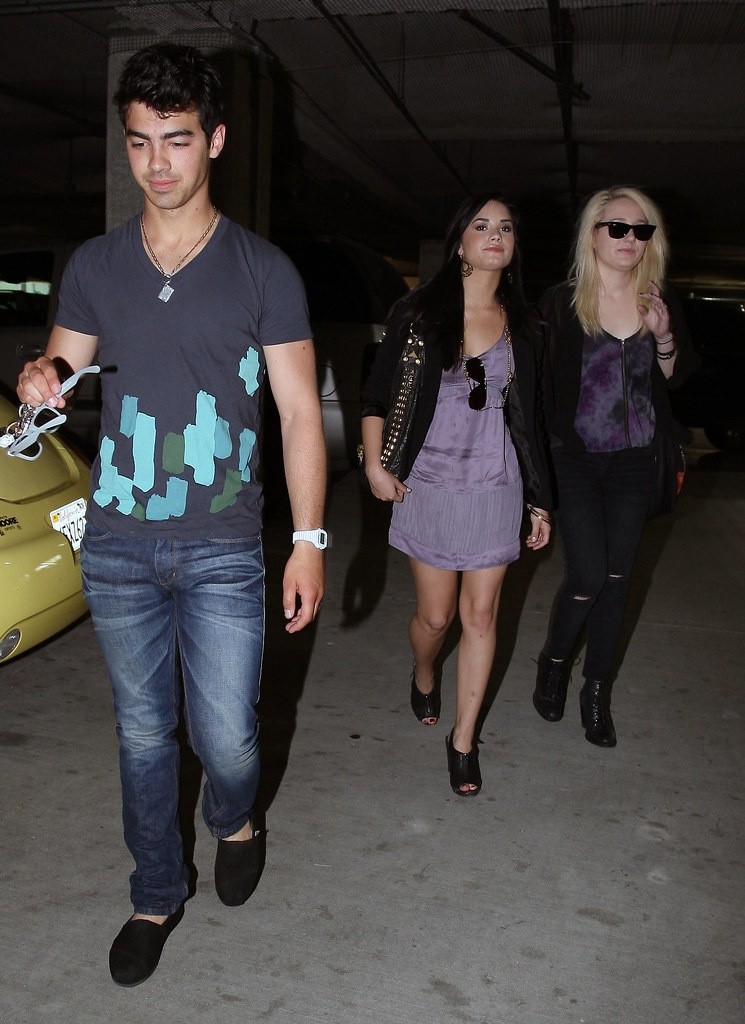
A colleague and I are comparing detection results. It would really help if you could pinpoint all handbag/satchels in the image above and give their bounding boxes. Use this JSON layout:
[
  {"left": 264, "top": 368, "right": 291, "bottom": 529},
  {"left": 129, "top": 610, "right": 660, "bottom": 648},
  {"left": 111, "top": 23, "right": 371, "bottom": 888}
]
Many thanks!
[{"left": 357, "top": 309, "right": 428, "bottom": 488}]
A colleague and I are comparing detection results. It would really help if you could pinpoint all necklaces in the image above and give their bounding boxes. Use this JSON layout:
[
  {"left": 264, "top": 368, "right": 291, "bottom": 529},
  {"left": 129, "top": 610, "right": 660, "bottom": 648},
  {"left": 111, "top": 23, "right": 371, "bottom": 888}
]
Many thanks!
[
  {"left": 460, "top": 302, "right": 512, "bottom": 412},
  {"left": 140, "top": 203, "right": 219, "bottom": 303}
]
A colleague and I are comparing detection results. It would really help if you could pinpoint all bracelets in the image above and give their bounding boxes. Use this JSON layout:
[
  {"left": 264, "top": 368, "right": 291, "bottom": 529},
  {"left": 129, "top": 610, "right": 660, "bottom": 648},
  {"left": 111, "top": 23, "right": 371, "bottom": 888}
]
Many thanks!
[
  {"left": 654, "top": 333, "right": 676, "bottom": 360},
  {"left": 529, "top": 505, "right": 550, "bottom": 524}
]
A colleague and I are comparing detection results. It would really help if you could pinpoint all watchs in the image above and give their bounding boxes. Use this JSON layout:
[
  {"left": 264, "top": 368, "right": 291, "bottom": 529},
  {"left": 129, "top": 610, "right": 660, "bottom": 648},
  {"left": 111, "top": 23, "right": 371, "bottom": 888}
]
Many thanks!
[{"left": 292, "top": 529, "right": 329, "bottom": 550}]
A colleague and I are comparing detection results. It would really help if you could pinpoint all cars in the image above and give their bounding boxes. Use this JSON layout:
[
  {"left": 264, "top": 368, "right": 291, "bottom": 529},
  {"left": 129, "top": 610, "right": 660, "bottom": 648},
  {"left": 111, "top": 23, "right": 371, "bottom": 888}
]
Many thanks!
[
  {"left": 1, "top": 395, "right": 93, "bottom": 664},
  {"left": 539, "top": 275, "right": 744, "bottom": 450}
]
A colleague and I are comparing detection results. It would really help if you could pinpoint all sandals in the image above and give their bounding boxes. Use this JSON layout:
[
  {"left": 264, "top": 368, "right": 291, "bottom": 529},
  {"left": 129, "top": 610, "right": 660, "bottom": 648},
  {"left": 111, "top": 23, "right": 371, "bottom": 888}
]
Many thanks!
[
  {"left": 410, "top": 663, "right": 441, "bottom": 726},
  {"left": 445, "top": 726, "right": 483, "bottom": 800}
]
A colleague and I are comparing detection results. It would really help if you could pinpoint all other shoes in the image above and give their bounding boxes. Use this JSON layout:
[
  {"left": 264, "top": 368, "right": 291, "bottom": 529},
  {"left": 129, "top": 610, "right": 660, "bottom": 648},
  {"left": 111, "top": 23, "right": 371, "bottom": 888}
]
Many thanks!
[
  {"left": 533, "top": 650, "right": 574, "bottom": 723},
  {"left": 579, "top": 680, "right": 618, "bottom": 749},
  {"left": 109, "top": 901, "right": 186, "bottom": 989},
  {"left": 214, "top": 816, "right": 267, "bottom": 907}
]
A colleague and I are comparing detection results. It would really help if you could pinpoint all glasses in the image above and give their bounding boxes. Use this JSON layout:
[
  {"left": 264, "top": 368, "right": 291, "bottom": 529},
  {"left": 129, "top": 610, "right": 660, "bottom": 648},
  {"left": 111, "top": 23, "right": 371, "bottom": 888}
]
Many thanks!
[
  {"left": 7, "top": 363, "right": 119, "bottom": 461},
  {"left": 595, "top": 221, "right": 657, "bottom": 242},
  {"left": 465, "top": 357, "right": 488, "bottom": 411}
]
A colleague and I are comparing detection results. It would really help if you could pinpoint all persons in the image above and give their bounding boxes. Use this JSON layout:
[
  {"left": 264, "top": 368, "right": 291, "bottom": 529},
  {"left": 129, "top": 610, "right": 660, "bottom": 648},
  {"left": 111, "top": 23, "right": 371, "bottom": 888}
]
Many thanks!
[
  {"left": 17, "top": 41, "right": 330, "bottom": 987},
  {"left": 359, "top": 189, "right": 554, "bottom": 798},
  {"left": 538, "top": 185, "right": 686, "bottom": 749}
]
